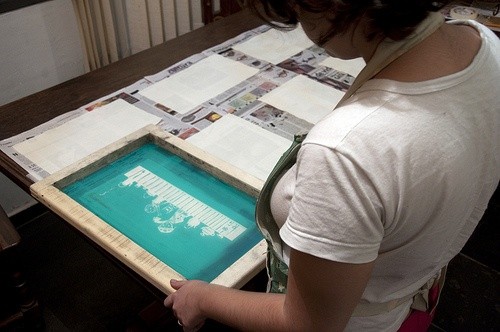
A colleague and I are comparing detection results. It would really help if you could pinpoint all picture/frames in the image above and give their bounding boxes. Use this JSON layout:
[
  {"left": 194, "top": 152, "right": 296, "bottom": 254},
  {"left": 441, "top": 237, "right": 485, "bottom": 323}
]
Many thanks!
[{"left": 30, "top": 122, "right": 270, "bottom": 302}]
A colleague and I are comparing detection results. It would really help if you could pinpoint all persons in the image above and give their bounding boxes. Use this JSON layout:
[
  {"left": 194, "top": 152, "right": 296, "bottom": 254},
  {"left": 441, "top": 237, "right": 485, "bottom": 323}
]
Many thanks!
[{"left": 165, "top": 0, "right": 500, "bottom": 332}]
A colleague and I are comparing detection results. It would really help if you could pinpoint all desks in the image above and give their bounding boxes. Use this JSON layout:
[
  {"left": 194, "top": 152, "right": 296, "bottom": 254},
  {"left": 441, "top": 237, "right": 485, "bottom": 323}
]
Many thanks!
[{"left": 0, "top": 1, "right": 368, "bottom": 330}]
